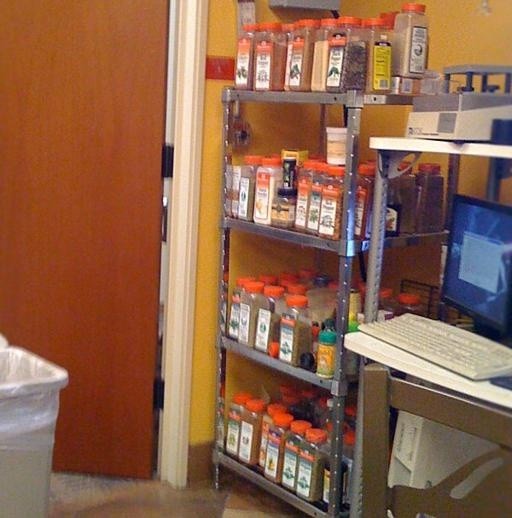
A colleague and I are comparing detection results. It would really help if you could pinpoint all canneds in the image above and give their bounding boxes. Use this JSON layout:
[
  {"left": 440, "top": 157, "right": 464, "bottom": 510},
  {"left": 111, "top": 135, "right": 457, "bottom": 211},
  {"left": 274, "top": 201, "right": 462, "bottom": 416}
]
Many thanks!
[{"left": 271, "top": 186, "right": 296, "bottom": 230}]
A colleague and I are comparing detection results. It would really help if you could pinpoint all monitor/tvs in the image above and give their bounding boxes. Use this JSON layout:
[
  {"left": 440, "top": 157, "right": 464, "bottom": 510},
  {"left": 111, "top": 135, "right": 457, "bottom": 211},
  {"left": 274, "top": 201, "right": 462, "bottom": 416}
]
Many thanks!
[{"left": 441, "top": 194, "right": 512, "bottom": 340}]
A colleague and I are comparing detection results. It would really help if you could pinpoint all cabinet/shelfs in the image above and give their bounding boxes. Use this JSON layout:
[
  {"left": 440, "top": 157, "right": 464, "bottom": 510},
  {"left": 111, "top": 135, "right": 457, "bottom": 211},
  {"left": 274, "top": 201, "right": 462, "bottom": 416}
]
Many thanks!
[
  {"left": 210, "top": 86, "right": 460, "bottom": 517},
  {"left": 351, "top": 135, "right": 511, "bottom": 517}
]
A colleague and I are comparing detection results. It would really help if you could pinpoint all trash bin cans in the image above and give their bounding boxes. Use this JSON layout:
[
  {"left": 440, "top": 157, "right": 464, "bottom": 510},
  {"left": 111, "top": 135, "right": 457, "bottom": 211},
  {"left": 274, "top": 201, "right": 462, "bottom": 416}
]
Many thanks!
[{"left": 1, "top": 345, "right": 70, "bottom": 518}]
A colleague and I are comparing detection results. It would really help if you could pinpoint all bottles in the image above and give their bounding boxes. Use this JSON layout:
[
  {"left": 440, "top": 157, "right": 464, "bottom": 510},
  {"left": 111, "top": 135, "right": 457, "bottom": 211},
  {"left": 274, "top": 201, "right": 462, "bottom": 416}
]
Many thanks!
[
  {"left": 225, "top": 270, "right": 424, "bottom": 510},
  {"left": 236, "top": 2, "right": 445, "bottom": 97},
  {"left": 228, "top": 127, "right": 445, "bottom": 239}
]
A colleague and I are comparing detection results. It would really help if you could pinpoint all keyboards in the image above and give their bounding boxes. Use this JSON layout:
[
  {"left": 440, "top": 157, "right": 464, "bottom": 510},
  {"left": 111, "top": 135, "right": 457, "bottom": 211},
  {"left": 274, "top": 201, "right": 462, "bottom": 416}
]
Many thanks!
[{"left": 358, "top": 312, "right": 512, "bottom": 380}]
